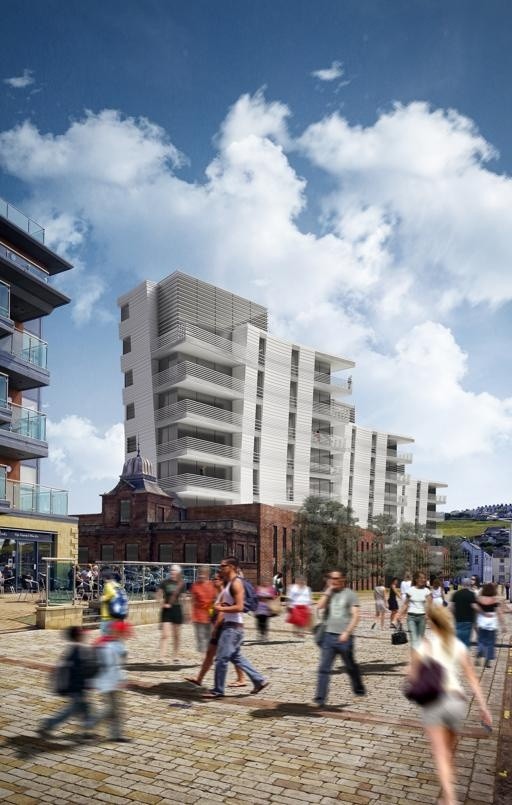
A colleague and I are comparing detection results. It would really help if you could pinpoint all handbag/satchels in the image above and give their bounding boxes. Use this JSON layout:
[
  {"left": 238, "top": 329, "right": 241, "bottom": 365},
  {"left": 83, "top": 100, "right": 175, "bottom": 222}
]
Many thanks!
[
  {"left": 402, "top": 659, "right": 447, "bottom": 707},
  {"left": 311, "top": 598, "right": 331, "bottom": 646},
  {"left": 390, "top": 621, "right": 408, "bottom": 645}
]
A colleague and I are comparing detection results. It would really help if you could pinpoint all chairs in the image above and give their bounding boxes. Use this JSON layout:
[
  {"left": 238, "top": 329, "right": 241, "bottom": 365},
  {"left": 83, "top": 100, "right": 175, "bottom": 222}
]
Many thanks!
[{"left": 0, "top": 565, "right": 184, "bottom": 602}]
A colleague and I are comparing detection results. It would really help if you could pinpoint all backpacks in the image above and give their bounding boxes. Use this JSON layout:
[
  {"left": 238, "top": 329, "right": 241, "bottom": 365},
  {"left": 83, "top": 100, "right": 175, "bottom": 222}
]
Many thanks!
[
  {"left": 109, "top": 587, "right": 130, "bottom": 620},
  {"left": 242, "top": 579, "right": 258, "bottom": 613}
]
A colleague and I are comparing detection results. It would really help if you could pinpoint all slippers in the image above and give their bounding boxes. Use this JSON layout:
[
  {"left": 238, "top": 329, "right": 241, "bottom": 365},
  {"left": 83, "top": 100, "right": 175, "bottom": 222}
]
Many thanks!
[
  {"left": 199, "top": 691, "right": 224, "bottom": 699},
  {"left": 184, "top": 677, "right": 201, "bottom": 687},
  {"left": 227, "top": 682, "right": 246, "bottom": 687},
  {"left": 250, "top": 682, "right": 270, "bottom": 695}
]
